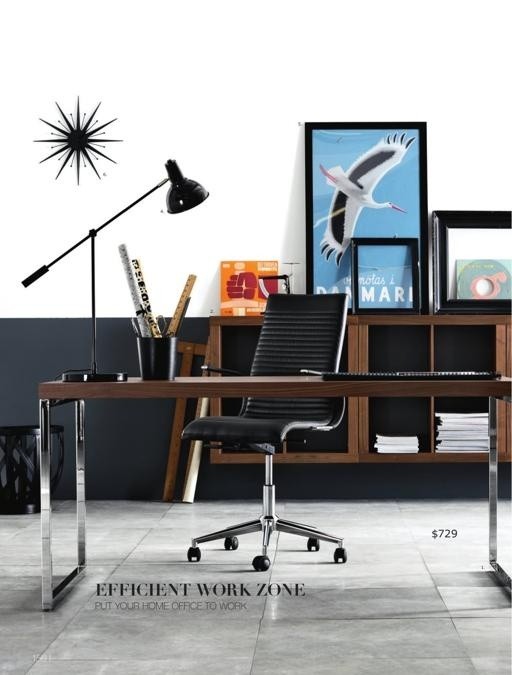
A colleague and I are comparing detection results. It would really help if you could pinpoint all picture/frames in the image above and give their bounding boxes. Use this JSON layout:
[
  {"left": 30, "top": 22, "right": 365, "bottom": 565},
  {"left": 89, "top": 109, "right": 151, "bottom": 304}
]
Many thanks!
[{"left": 304, "top": 119, "right": 512, "bottom": 316}]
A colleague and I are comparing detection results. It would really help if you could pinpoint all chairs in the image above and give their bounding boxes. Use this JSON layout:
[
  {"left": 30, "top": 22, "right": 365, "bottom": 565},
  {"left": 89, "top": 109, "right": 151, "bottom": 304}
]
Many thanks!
[{"left": 180, "top": 294, "right": 350, "bottom": 571}]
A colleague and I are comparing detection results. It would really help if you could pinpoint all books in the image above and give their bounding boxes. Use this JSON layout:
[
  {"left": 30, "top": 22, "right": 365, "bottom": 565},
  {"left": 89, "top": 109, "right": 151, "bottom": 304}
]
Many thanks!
[
  {"left": 433, "top": 412, "right": 490, "bottom": 453},
  {"left": 373, "top": 433, "right": 421, "bottom": 454}
]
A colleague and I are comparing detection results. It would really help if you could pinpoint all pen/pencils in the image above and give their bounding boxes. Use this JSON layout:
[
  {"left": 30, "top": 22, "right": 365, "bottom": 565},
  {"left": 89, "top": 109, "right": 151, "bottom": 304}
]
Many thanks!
[{"left": 130, "top": 317, "right": 140, "bottom": 337}]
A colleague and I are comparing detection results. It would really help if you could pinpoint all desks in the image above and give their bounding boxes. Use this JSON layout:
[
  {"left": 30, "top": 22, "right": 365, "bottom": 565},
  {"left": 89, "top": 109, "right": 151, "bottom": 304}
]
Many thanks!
[{"left": 35, "top": 373, "right": 511, "bottom": 611}]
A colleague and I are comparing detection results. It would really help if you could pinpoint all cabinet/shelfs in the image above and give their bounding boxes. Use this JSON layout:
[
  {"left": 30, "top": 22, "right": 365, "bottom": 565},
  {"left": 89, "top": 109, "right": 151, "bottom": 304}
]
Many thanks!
[{"left": 208, "top": 312, "right": 511, "bottom": 463}]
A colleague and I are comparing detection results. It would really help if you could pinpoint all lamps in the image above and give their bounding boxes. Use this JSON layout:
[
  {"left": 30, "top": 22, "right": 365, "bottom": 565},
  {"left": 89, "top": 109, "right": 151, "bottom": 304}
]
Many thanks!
[{"left": 22, "top": 157, "right": 210, "bottom": 381}]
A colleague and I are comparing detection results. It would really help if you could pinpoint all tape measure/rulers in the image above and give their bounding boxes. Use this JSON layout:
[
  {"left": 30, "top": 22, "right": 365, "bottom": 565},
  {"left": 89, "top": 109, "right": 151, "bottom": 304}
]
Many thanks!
[{"left": 167, "top": 274, "right": 196, "bottom": 336}]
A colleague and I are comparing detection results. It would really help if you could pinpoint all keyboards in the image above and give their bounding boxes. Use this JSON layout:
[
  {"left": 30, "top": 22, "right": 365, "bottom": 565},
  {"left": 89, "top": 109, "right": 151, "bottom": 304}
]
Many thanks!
[{"left": 323, "top": 371, "right": 497, "bottom": 380}]
